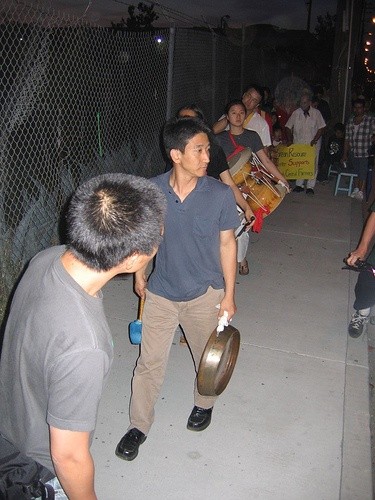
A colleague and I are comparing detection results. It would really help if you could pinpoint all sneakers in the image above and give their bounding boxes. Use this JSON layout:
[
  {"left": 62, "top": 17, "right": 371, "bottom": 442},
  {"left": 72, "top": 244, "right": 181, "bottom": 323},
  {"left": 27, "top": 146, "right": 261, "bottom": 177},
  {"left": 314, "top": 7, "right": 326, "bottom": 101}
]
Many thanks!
[{"left": 347, "top": 310, "right": 370, "bottom": 338}]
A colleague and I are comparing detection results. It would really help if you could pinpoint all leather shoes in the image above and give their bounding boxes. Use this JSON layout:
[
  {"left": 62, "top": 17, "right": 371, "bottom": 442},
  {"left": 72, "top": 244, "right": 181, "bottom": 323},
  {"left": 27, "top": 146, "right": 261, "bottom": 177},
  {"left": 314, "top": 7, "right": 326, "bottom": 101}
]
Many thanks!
[
  {"left": 116, "top": 428, "right": 146, "bottom": 460},
  {"left": 186, "top": 406, "right": 213, "bottom": 431}
]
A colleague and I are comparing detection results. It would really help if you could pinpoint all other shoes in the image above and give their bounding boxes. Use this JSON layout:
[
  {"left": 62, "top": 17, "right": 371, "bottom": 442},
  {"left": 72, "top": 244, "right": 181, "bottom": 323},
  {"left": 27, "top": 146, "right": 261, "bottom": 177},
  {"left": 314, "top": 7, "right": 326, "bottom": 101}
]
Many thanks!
[
  {"left": 293, "top": 187, "right": 303, "bottom": 192},
  {"left": 306, "top": 188, "right": 314, "bottom": 195},
  {"left": 351, "top": 189, "right": 364, "bottom": 200}
]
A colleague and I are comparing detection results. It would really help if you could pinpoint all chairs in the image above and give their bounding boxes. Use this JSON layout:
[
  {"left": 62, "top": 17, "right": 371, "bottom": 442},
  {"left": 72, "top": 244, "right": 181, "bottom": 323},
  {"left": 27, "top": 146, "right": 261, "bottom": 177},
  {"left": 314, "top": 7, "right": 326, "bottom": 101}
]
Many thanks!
[{"left": 324, "top": 160, "right": 357, "bottom": 195}]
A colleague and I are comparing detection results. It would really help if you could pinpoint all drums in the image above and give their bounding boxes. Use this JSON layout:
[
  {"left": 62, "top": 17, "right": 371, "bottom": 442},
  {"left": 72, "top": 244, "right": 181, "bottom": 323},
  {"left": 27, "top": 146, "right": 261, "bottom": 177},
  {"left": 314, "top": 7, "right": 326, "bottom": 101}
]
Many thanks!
[{"left": 228, "top": 148, "right": 287, "bottom": 219}]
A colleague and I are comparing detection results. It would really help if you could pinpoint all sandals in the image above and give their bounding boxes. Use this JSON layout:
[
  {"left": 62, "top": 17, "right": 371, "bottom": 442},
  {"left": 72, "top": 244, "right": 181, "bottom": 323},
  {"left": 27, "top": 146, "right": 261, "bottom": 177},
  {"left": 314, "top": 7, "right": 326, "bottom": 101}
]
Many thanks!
[{"left": 238, "top": 261, "right": 248, "bottom": 274}]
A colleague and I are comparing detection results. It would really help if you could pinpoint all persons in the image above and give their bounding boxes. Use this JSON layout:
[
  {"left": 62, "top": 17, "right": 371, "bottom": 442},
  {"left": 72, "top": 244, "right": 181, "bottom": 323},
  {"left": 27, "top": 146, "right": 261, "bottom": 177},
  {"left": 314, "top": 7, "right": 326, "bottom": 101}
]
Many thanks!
[
  {"left": 257, "top": 108, "right": 272, "bottom": 137},
  {"left": 347, "top": 200, "right": 375, "bottom": 338},
  {"left": 318, "top": 122, "right": 344, "bottom": 184},
  {"left": 311, "top": 86, "right": 331, "bottom": 124},
  {"left": 116, "top": 116, "right": 241, "bottom": 461},
  {"left": 215, "top": 100, "right": 292, "bottom": 275},
  {"left": 178, "top": 102, "right": 255, "bottom": 231},
  {"left": 213, "top": 86, "right": 272, "bottom": 156},
  {"left": 0, "top": 172, "right": 166, "bottom": 499},
  {"left": 285, "top": 93, "right": 326, "bottom": 195},
  {"left": 343, "top": 98, "right": 375, "bottom": 202}
]
